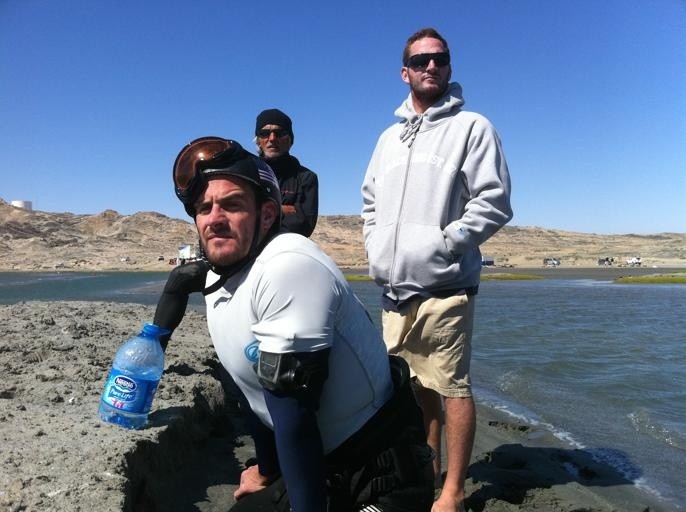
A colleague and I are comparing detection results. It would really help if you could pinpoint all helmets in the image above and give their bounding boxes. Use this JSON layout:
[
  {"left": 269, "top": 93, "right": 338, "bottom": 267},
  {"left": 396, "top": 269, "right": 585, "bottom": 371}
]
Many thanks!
[{"left": 185, "top": 151, "right": 282, "bottom": 241}]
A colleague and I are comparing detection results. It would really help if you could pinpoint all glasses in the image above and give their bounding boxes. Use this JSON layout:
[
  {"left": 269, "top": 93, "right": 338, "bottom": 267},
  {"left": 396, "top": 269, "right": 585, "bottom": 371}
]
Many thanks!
[
  {"left": 257, "top": 128, "right": 287, "bottom": 138},
  {"left": 173, "top": 136, "right": 243, "bottom": 206},
  {"left": 407, "top": 53, "right": 450, "bottom": 69}
]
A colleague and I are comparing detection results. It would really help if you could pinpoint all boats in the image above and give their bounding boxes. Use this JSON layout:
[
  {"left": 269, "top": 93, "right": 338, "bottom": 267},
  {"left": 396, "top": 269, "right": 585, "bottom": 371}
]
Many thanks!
[
  {"left": 346, "top": 274, "right": 547, "bottom": 281},
  {"left": 613, "top": 276, "right": 686, "bottom": 284}
]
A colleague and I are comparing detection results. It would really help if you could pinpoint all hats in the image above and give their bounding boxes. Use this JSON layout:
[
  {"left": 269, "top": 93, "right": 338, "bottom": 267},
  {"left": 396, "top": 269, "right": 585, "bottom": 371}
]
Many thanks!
[{"left": 255, "top": 109, "right": 294, "bottom": 144}]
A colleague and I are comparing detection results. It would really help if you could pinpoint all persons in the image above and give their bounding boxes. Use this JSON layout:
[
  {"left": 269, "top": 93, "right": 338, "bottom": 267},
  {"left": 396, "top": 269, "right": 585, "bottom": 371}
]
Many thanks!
[
  {"left": 142, "top": 107, "right": 320, "bottom": 353},
  {"left": 171, "top": 134, "right": 438, "bottom": 511},
  {"left": 359, "top": 27, "right": 516, "bottom": 511}
]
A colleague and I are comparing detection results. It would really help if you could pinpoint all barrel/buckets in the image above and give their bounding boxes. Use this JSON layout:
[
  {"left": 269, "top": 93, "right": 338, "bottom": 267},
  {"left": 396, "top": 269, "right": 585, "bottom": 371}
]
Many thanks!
[{"left": 97, "top": 322, "right": 171, "bottom": 429}]
